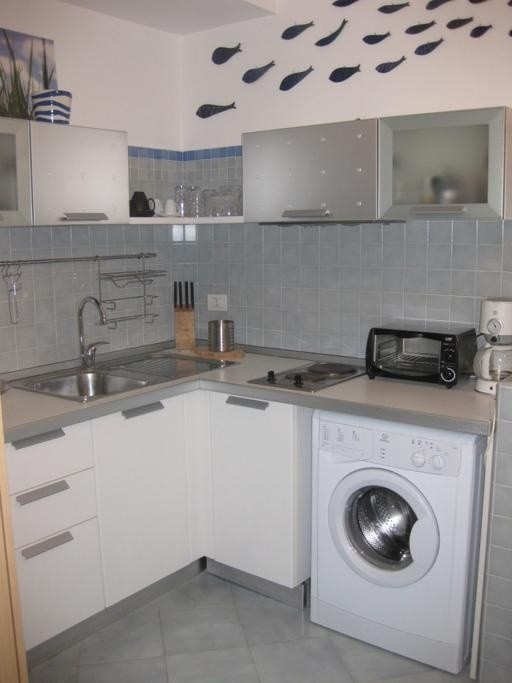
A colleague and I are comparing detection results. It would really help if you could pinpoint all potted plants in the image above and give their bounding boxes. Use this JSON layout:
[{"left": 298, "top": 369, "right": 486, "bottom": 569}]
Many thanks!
[{"left": 31, "top": 63, "right": 72, "bottom": 125}]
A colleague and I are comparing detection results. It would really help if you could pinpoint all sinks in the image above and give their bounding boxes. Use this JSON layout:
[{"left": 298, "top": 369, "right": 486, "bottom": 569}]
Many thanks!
[
  {"left": 106, "top": 352, "right": 237, "bottom": 384},
  {"left": 6, "top": 367, "right": 151, "bottom": 404}
]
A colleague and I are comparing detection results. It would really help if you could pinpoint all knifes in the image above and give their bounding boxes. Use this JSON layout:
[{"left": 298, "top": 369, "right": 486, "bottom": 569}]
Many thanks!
[
  {"left": 174, "top": 281, "right": 177, "bottom": 308},
  {"left": 185, "top": 282, "right": 188, "bottom": 307},
  {"left": 179, "top": 282, "right": 182, "bottom": 307},
  {"left": 190, "top": 282, "right": 194, "bottom": 308}
]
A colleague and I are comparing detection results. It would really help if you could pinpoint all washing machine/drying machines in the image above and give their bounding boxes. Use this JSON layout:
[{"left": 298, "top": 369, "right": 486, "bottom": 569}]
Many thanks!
[{"left": 310, "top": 409, "right": 487, "bottom": 674}]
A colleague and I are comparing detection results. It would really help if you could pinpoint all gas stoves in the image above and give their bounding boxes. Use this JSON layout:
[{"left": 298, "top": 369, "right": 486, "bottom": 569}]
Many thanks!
[{"left": 246, "top": 362, "right": 368, "bottom": 392}]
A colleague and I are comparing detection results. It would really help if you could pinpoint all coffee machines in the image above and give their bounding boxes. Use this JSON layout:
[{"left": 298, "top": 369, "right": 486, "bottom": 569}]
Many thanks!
[{"left": 472, "top": 297, "right": 512, "bottom": 395}]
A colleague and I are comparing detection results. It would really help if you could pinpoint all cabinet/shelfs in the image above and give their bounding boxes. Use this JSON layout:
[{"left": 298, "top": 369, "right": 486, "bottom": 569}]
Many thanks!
[
  {"left": 3, "top": 420, "right": 106, "bottom": 652},
  {"left": 204, "top": 392, "right": 311, "bottom": 589},
  {"left": 97, "top": 253, "right": 168, "bottom": 329},
  {"left": 1, "top": 118, "right": 33, "bottom": 228},
  {"left": 242, "top": 118, "right": 377, "bottom": 223},
  {"left": 93, "top": 388, "right": 204, "bottom": 609},
  {"left": 29, "top": 120, "right": 130, "bottom": 226}
]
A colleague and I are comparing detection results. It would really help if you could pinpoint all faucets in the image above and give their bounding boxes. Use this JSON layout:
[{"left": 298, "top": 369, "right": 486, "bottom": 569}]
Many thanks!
[{"left": 76, "top": 295, "right": 110, "bottom": 368}]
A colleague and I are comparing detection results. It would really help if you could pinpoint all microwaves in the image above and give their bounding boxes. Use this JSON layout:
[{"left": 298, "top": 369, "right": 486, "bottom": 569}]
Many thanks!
[{"left": 365, "top": 320, "right": 476, "bottom": 389}]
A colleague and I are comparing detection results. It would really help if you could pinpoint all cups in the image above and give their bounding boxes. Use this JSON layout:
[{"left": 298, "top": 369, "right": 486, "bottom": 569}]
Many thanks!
[
  {"left": 208, "top": 320, "right": 235, "bottom": 352},
  {"left": 203, "top": 184, "right": 243, "bottom": 216},
  {"left": 149, "top": 199, "right": 164, "bottom": 216},
  {"left": 176, "top": 185, "right": 188, "bottom": 218},
  {"left": 164, "top": 199, "right": 181, "bottom": 215},
  {"left": 189, "top": 184, "right": 201, "bottom": 218},
  {"left": 130, "top": 191, "right": 155, "bottom": 213}
]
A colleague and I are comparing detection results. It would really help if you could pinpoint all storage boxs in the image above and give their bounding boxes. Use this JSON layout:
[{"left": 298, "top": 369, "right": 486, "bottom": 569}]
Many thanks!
[{"left": 378, "top": 106, "right": 512, "bottom": 222}]
